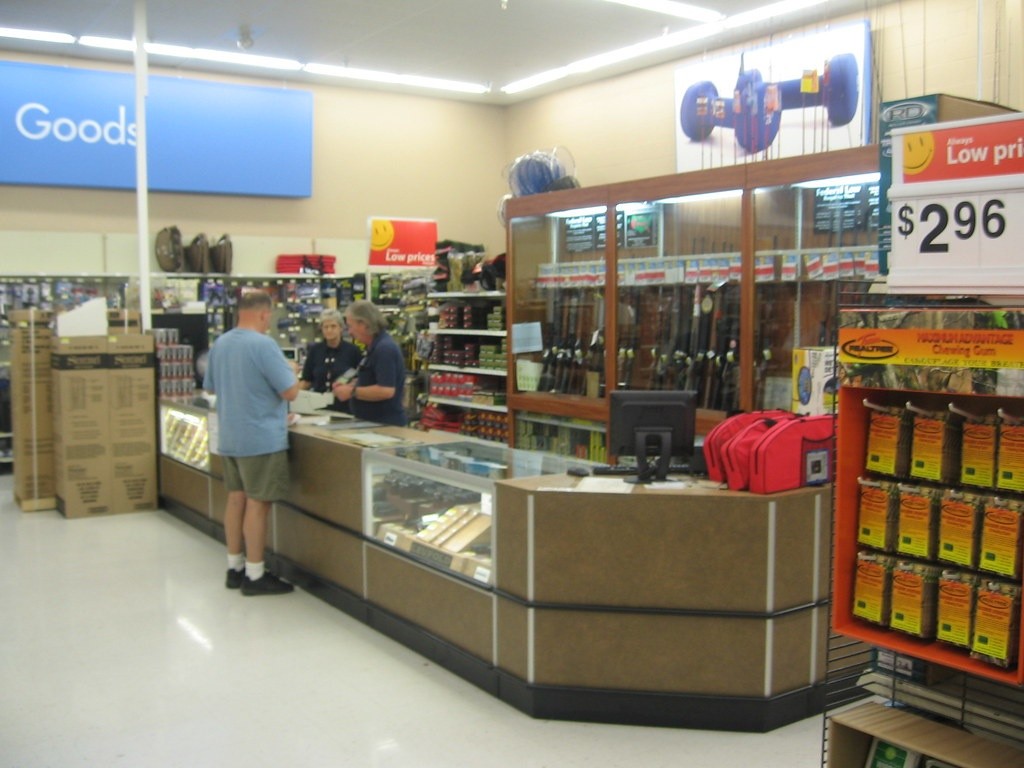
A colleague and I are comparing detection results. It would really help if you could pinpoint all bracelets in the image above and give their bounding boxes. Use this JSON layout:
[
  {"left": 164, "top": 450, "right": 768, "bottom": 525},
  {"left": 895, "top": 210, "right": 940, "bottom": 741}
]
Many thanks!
[{"left": 352, "top": 387, "right": 356, "bottom": 399}]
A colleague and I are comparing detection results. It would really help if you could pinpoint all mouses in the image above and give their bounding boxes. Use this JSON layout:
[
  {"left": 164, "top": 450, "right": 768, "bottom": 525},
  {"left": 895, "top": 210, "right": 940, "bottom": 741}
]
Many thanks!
[{"left": 567, "top": 466, "right": 589, "bottom": 476}]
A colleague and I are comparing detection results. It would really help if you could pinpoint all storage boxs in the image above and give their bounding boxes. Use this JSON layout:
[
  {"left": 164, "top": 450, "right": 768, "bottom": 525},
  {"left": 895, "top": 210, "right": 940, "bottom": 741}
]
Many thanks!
[
  {"left": 6, "top": 309, "right": 144, "bottom": 513},
  {"left": 827, "top": 703, "right": 1024, "bottom": 768},
  {"left": 48, "top": 333, "right": 160, "bottom": 519}
]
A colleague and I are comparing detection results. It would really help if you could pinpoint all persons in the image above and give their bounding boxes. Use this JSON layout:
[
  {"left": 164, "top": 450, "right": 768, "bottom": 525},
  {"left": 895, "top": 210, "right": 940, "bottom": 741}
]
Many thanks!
[
  {"left": 203, "top": 291, "right": 300, "bottom": 596},
  {"left": 332, "top": 300, "right": 405, "bottom": 427},
  {"left": 299, "top": 308, "right": 362, "bottom": 415}
]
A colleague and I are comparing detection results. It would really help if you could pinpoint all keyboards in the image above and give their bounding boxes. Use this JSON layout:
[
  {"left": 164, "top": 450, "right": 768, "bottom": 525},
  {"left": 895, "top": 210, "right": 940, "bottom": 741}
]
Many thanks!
[{"left": 592, "top": 465, "right": 695, "bottom": 475}]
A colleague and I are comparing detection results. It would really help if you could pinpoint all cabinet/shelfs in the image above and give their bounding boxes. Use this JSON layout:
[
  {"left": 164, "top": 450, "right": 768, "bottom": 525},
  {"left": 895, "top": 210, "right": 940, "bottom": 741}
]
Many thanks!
[
  {"left": 825, "top": 307, "right": 1024, "bottom": 687},
  {"left": 0, "top": 272, "right": 422, "bottom": 465},
  {"left": 424, "top": 288, "right": 509, "bottom": 414},
  {"left": 510, "top": 142, "right": 882, "bottom": 469},
  {"left": 157, "top": 394, "right": 832, "bottom": 734}
]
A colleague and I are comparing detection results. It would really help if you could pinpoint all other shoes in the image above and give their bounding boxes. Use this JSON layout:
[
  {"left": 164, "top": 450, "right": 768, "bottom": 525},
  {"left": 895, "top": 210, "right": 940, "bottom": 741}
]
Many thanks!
[
  {"left": 242, "top": 571, "right": 294, "bottom": 595},
  {"left": 227, "top": 566, "right": 278, "bottom": 589}
]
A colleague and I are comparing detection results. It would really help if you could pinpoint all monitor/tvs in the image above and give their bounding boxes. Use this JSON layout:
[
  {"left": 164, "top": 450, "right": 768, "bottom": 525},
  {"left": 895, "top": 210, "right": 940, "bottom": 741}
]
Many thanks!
[{"left": 609, "top": 389, "right": 698, "bottom": 485}]
